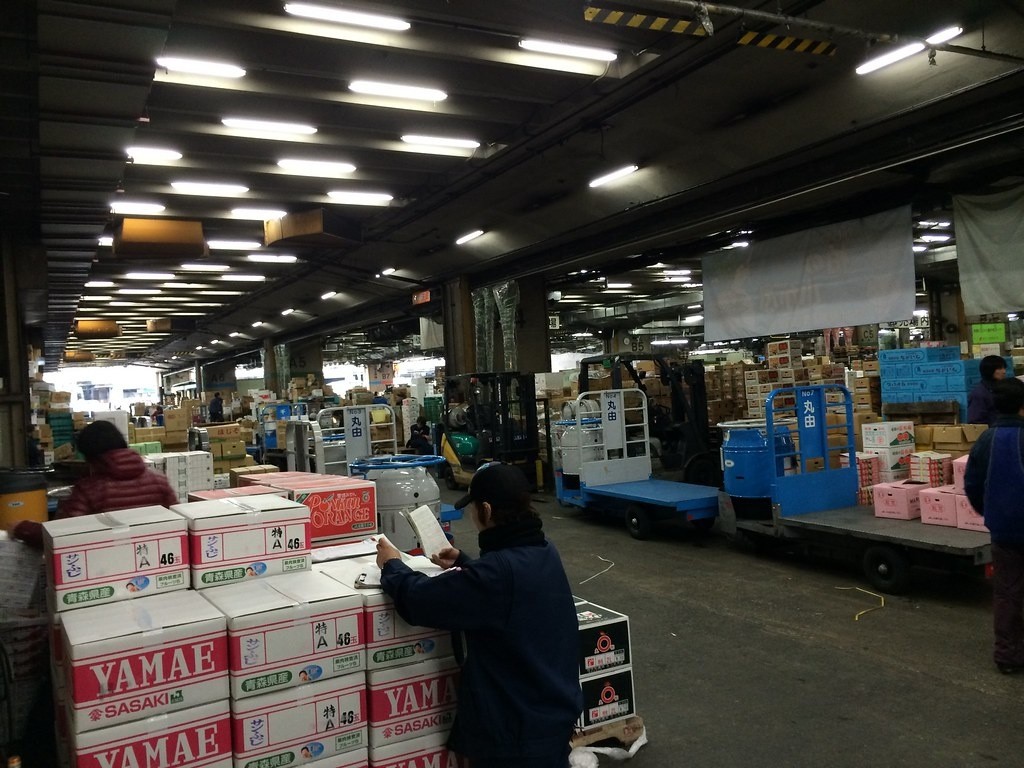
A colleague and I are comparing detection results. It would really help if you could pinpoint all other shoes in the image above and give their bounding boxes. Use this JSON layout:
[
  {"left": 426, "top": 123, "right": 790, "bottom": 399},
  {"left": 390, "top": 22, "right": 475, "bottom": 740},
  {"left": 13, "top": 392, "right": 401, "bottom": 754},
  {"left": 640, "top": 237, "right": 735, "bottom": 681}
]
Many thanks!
[{"left": 997, "top": 661, "right": 1024, "bottom": 675}]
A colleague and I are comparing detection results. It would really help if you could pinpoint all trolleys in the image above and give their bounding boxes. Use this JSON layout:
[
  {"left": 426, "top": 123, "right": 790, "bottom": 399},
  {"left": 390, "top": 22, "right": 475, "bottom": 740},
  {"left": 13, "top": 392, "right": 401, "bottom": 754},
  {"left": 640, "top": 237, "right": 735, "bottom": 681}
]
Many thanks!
[
  {"left": 733, "top": 383, "right": 993, "bottom": 598},
  {"left": 316, "top": 403, "right": 399, "bottom": 476},
  {"left": 554, "top": 385, "right": 722, "bottom": 541}
]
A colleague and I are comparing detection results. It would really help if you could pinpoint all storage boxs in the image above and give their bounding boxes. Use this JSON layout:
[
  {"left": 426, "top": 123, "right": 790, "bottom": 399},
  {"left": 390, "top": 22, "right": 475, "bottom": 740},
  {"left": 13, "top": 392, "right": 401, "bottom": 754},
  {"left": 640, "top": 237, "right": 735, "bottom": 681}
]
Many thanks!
[
  {"left": 29, "top": 373, "right": 634, "bottom": 768},
  {"left": 535, "top": 338, "right": 1024, "bottom": 533}
]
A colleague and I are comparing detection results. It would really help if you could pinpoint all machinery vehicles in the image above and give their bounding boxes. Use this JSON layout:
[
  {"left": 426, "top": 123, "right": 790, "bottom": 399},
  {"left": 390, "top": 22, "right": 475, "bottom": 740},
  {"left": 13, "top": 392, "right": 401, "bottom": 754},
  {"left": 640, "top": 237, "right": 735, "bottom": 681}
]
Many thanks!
[{"left": 438, "top": 371, "right": 553, "bottom": 504}]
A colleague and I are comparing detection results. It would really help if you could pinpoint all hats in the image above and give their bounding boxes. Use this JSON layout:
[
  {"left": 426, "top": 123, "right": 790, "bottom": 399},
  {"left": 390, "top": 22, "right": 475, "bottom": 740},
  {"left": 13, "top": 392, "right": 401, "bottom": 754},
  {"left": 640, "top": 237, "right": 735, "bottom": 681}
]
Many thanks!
[{"left": 455, "top": 462, "right": 533, "bottom": 510}]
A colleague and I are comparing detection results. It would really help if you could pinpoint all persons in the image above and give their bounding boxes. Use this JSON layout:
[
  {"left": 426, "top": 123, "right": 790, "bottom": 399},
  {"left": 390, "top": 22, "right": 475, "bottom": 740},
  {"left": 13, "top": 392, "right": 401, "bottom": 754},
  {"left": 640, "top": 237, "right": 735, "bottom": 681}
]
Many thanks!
[
  {"left": 376, "top": 464, "right": 584, "bottom": 768},
  {"left": 151, "top": 406, "right": 163, "bottom": 420},
  {"left": 374, "top": 392, "right": 377, "bottom": 396},
  {"left": 7, "top": 420, "right": 180, "bottom": 552},
  {"left": 332, "top": 410, "right": 344, "bottom": 434},
  {"left": 407, "top": 416, "right": 432, "bottom": 455},
  {"left": 964, "top": 377, "right": 1024, "bottom": 676},
  {"left": 966, "top": 355, "right": 1008, "bottom": 424},
  {"left": 210, "top": 392, "right": 224, "bottom": 422}
]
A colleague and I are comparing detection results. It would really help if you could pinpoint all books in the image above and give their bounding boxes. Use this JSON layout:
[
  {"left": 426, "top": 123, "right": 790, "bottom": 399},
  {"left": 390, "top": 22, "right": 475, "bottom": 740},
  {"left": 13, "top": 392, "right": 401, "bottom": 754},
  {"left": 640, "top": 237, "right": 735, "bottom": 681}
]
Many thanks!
[{"left": 398, "top": 505, "right": 454, "bottom": 559}]
